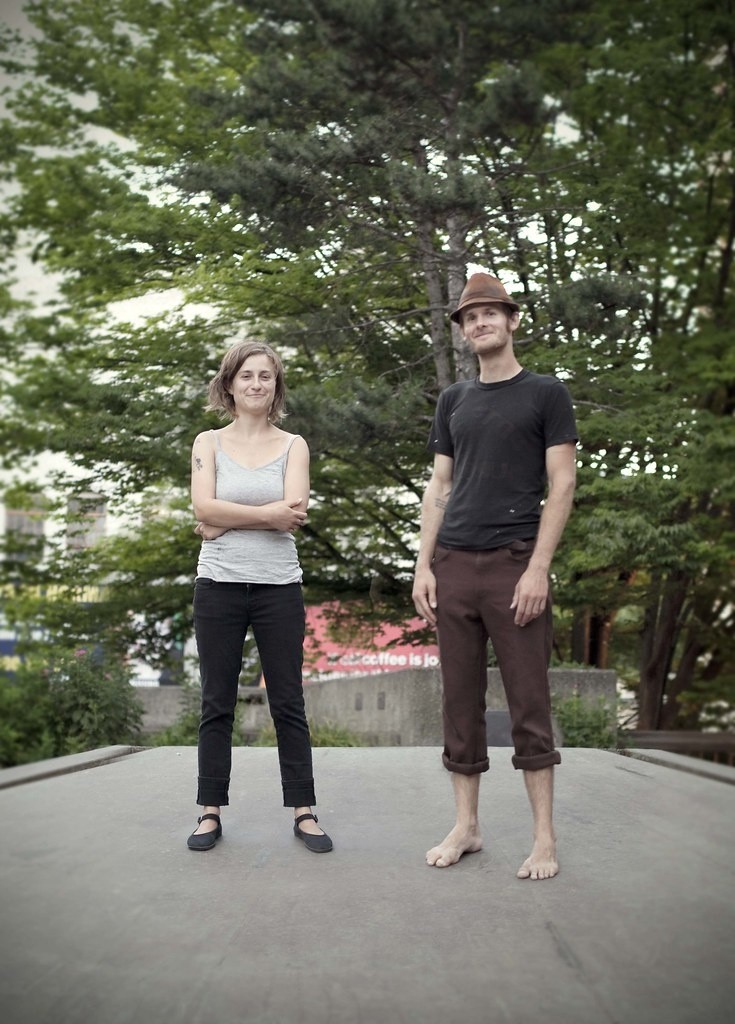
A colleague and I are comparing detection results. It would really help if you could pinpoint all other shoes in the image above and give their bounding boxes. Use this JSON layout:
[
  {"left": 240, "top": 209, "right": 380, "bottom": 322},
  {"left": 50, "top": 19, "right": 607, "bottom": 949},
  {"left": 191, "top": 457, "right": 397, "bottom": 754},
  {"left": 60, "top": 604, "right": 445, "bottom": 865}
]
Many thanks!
[
  {"left": 293, "top": 813, "right": 333, "bottom": 853},
  {"left": 187, "top": 814, "right": 222, "bottom": 851}
]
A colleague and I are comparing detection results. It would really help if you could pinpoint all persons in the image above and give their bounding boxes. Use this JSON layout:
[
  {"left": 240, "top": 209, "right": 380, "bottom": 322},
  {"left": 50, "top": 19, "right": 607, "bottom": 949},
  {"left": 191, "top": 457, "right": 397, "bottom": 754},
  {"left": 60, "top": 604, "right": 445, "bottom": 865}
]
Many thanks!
[
  {"left": 187, "top": 340, "right": 332, "bottom": 853},
  {"left": 412, "top": 273, "right": 578, "bottom": 879}
]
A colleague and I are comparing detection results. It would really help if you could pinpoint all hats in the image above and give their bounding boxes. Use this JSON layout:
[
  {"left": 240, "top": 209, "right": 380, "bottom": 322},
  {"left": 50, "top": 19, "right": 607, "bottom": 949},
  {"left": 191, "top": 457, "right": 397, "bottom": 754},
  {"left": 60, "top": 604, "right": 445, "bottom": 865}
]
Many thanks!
[{"left": 449, "top": 272, "right": 520, "bottom": 324}]
